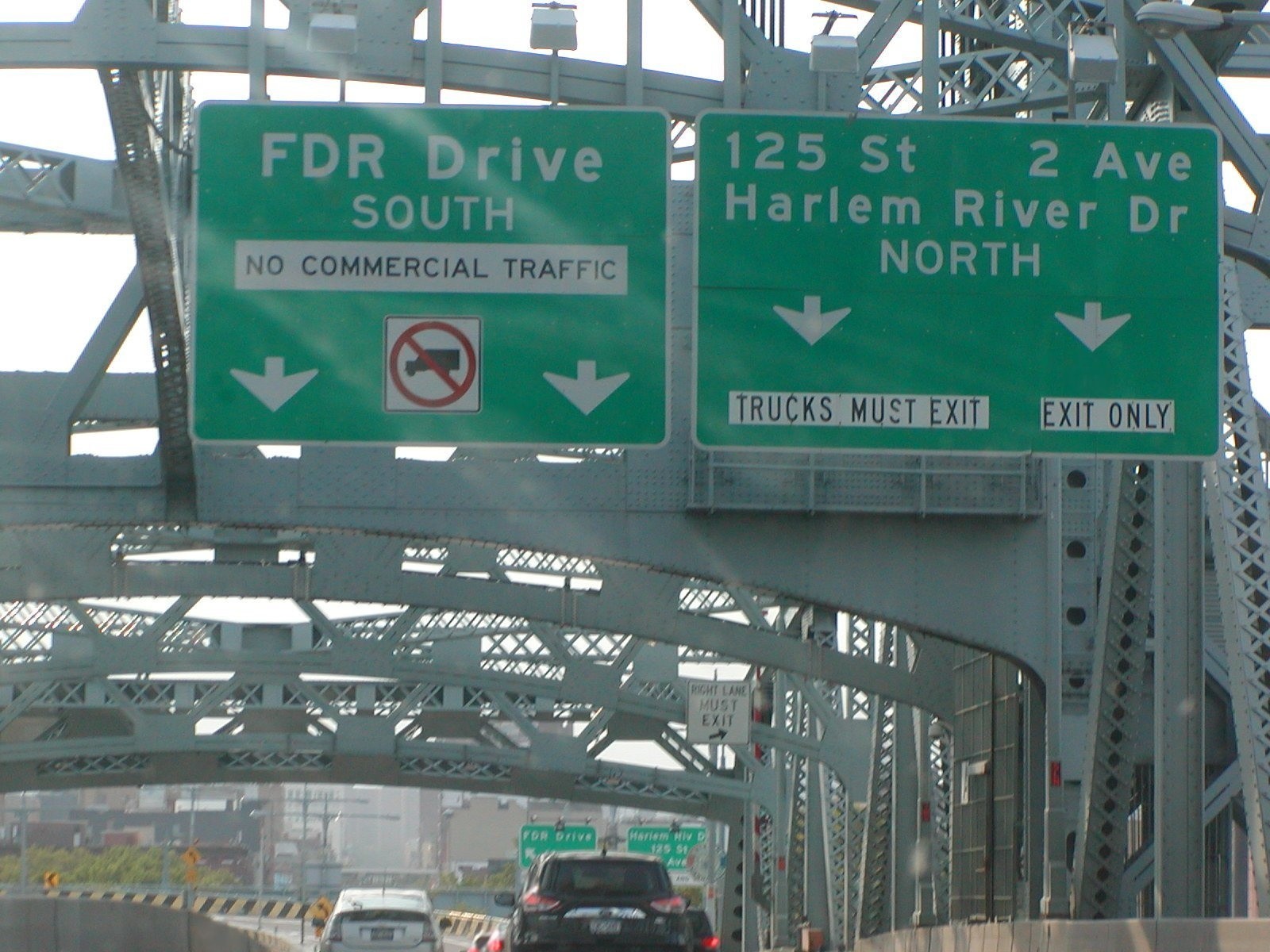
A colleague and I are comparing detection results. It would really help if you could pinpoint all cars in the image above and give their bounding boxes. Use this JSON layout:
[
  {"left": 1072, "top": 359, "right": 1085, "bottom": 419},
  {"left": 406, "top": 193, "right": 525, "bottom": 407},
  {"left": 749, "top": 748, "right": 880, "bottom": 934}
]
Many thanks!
[
  {"left": 311, "top": 889, "right": 453, "bottom": 952},
  {"left": 687, "top": 909, "right": 721, "bottom": 952}
]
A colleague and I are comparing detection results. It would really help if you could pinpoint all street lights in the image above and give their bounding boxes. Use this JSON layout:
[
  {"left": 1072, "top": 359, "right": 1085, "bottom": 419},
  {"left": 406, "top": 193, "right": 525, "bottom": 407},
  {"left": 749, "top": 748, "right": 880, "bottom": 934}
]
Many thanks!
[
  {"left": 248, "top": 792, "right": 402, "bottom": 897},
  {"left": 239, "top": 779, "right": 371, "bottom": 901}
]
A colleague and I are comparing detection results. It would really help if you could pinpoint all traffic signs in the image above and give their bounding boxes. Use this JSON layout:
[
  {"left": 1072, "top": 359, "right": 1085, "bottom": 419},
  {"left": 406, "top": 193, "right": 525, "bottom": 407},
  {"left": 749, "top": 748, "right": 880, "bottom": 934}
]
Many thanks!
[
  {"left": 627, "top": 825, "right": 708, "bottom": 871},
  {"left": 690, "top": 109, "right": 1224, "bottom": 461},
  {"left": 685, "top": 679, "right": 755, "bottom": 747},
  {"left": 519, "top": 823, "right": 599, "bottom": 868},
  {"left": 185, "top": 97, "right": 673, "bottom": 450}
]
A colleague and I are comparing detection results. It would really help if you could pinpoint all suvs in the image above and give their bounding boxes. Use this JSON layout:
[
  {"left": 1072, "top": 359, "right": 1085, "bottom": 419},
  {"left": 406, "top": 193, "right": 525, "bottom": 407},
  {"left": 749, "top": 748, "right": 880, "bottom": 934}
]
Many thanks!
[{"left": 469, "top": 848, "right": 695, "bottom": 952}]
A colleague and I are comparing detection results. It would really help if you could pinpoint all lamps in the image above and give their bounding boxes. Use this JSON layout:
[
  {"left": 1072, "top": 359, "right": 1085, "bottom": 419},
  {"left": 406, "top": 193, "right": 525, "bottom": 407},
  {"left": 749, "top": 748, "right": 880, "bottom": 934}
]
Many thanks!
[
  {"left": 1069, "top": 18, "right": 1118, "bottom": 84},
  {"left": 306, "top": 13, "right": 357, "bottom": 54},
  {"left": 530, "top": 9, "right": 577, "bottom": 50},
  {"left": 809, "top": 35, "right": 859, "bottom": 74}
]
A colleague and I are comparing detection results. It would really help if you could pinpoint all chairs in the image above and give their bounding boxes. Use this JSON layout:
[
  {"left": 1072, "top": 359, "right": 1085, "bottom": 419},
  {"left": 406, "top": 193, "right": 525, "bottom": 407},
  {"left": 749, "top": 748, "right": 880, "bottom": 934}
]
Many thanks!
[
  {"left": 624, "top": 869, "right": 648, "bottom": 894},
  {"left": 555, "top": 868, "right": 575, "bottom": 892}
]
